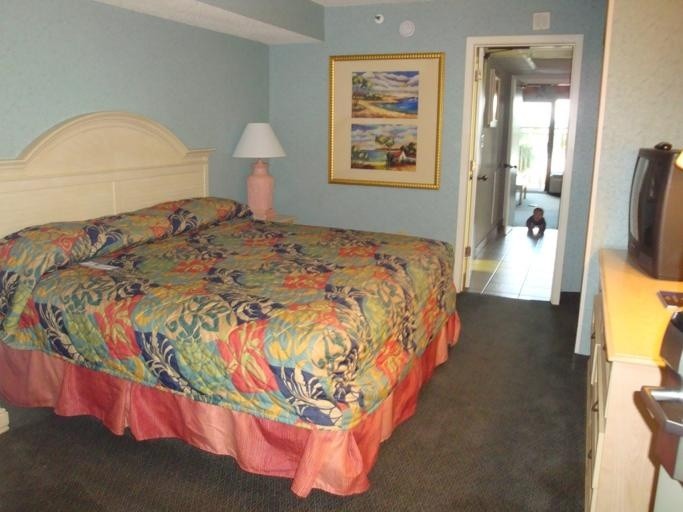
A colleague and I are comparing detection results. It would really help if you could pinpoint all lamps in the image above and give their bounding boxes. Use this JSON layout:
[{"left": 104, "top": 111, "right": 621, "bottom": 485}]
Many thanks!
[{"left": 233, "top": 122, "right": 285, "bottom": 218}]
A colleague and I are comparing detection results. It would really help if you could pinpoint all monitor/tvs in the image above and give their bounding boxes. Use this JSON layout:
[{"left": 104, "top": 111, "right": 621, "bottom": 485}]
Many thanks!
[{"left": 628, "top": 149, "right": 683, "bottom": 281}]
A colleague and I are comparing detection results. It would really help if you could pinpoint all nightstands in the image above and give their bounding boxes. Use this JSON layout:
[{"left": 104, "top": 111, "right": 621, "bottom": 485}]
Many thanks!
[{"left": 271, "top": 215, "right": 298, "bottom": 225}]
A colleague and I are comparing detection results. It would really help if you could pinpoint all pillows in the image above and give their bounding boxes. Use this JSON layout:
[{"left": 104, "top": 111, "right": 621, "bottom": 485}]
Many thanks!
[{"left": 1, "top": 197, "right": 242, "bottom": 331}]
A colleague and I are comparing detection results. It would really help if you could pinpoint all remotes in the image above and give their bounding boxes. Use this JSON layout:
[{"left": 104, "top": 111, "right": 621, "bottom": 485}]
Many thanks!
[{"left": 655, "top": 142, "right": 671, "bottom": 150}]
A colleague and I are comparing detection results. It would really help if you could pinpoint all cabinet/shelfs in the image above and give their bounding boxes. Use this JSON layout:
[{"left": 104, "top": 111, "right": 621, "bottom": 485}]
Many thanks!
[{"left": 585, "top": 248, "right": 683, "bottom": 512}]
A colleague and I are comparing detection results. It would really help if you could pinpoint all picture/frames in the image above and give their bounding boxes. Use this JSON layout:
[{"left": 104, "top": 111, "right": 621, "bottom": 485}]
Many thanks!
[{"left": 328, "top": 52, "right": 445, "bottom": 190}]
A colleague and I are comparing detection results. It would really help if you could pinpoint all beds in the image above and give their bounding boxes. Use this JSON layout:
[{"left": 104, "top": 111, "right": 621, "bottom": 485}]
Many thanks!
[{"left": 1, "top": 111, "right": 461, "bottom": 497}]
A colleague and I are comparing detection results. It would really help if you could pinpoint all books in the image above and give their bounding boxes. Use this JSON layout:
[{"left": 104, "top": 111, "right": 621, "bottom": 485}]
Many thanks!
[{"left": 657, "top": 291, "right": 683, "bottom": 308}]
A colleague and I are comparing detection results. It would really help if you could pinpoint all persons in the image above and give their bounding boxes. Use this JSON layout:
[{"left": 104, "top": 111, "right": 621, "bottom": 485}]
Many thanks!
[{"left": 526, "top": 207, "right": 546, "bottom": 232}]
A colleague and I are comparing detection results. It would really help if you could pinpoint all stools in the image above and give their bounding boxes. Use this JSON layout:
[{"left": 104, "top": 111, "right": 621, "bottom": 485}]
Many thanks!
[{"left": 516, "top": 182, "right": 527, "bottom": 205}]
